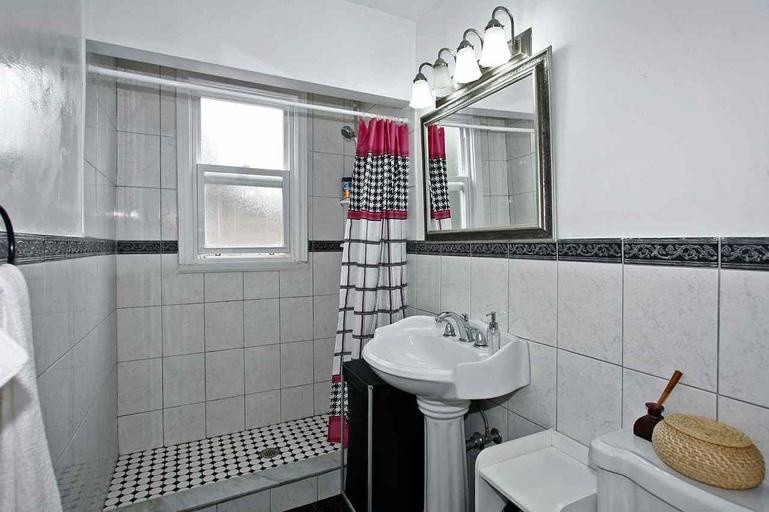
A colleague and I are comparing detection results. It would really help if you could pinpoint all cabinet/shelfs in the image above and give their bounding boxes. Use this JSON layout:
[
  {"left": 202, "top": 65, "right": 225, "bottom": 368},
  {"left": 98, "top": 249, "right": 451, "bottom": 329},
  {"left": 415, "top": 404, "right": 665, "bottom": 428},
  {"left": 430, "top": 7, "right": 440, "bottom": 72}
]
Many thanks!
[
  {"left": 340, "top": 359, "right": 425, "bottom": 512},
  {"left": 473, "top": 429, "right": 597, "bottom": 512}
]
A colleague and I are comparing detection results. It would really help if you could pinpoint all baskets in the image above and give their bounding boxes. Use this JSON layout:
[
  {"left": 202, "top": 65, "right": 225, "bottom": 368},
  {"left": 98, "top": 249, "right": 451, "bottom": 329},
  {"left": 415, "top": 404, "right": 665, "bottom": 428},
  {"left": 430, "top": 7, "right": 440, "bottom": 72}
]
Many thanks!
[{"left": 650, "top": 410, "right": 765, "bottom": 490}]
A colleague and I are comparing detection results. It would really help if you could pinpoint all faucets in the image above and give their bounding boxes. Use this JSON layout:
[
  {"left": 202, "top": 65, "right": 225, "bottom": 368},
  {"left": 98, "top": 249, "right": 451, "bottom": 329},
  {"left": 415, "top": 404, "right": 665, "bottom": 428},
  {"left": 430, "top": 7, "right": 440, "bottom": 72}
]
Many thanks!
[{"left": 435, "top": 311, "right": 472, "bottom": 341}]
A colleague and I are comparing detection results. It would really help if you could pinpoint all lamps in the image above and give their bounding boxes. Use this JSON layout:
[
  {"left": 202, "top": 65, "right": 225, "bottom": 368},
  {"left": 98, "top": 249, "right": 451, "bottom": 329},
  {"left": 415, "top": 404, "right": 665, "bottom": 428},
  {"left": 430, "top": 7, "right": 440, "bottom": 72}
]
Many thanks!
[{"left": 407, "top": 6, "right": 531, "bottom": 111}]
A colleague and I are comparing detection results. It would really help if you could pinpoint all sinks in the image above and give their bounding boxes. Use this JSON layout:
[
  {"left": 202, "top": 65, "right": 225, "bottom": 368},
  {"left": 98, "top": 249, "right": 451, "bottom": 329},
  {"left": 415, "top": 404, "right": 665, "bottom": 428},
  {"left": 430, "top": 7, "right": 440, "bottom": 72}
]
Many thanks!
[{"left": 361, "top": 316, "right": 530, "bottom": 401}]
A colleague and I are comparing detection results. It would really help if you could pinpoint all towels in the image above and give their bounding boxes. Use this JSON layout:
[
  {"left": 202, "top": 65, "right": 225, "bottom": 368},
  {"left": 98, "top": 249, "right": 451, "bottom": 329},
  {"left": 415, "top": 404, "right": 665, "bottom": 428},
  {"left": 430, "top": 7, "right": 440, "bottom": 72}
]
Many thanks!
[{"left": 0, "top": 262, "right": 66, "bottom": 511}]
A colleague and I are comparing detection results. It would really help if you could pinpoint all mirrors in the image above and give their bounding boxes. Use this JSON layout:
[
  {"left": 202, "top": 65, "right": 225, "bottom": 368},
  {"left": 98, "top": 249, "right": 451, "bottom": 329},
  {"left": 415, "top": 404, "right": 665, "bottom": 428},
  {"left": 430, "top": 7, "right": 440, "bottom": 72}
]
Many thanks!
[{"left": 420, "top": 45, "right": 552, "bottom": 241}]
